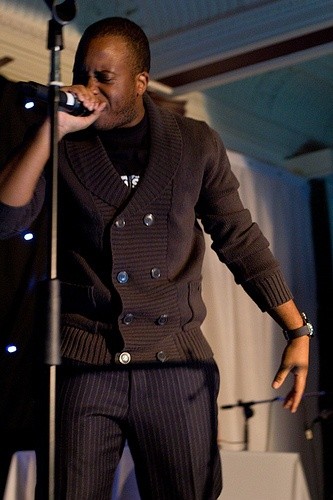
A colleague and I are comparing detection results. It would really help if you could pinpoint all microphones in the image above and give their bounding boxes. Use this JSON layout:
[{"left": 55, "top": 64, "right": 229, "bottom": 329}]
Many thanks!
[
  {"left": 312, "top": 409, "right": 333, "bottom": 423},
  {"left": 20, "top": 81, "right": 95, "bottom": 118}
]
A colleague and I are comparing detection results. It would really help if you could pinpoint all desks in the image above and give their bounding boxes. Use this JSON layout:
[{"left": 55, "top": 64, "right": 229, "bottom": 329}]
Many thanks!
[{"left": 3, "top": 450, "right": 311, "bottom": 500}]
